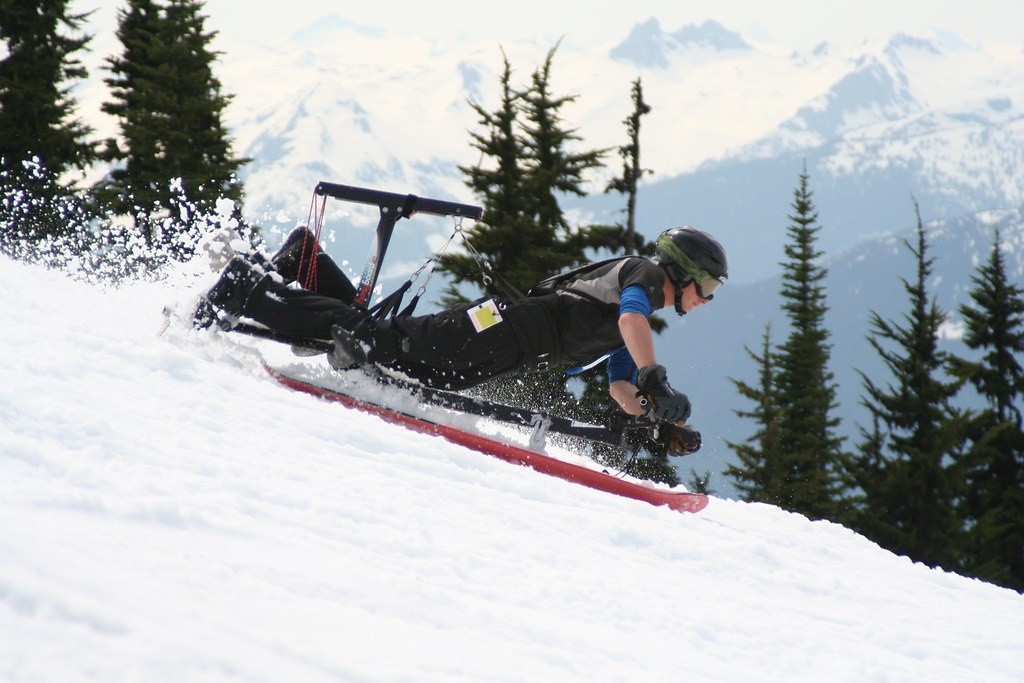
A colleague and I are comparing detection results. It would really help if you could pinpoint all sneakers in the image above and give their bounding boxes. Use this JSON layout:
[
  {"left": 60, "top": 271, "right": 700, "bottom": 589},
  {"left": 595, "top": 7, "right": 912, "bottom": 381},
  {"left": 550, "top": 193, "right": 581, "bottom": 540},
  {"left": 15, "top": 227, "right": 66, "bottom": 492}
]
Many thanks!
[
  {"left": 271, "top": 226, "right": 323, "bottom": 286},
  {"left": 193, "top": 255, "right": 269, "bottom": 333}
]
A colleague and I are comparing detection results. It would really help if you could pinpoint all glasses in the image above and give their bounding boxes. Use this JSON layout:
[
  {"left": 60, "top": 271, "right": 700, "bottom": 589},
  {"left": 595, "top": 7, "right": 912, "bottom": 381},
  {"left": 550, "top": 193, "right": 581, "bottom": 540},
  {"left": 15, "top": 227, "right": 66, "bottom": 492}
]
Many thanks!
[{"left": 657, "top": 233, "right": 724, "bottom": 301}]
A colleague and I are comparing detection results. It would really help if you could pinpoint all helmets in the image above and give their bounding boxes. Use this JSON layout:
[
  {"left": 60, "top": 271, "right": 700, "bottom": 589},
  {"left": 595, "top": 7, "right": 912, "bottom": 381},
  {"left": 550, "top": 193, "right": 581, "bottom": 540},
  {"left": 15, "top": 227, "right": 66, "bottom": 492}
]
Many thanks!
[{"left": 654, "top": 226, "right": 728, "bottom": 289}]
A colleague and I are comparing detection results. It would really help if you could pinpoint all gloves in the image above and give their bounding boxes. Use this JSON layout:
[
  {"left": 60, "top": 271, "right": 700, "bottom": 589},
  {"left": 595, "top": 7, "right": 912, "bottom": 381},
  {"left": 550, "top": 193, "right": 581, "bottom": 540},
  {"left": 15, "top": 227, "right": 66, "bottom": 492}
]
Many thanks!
[
  {"left": 638, "top": 364, "right": 692, "bottom": 425},
  {"left": 652, "top": 425, "right": 703, "bottom": 457}
]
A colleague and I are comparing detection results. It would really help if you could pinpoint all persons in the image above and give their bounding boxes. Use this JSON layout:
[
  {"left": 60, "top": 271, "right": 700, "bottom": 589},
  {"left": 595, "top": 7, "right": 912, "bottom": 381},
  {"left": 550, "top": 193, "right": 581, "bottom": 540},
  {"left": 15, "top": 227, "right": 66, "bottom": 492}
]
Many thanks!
[{"left": 193, "top": 227, "right": 728, "bottom": 457}]
